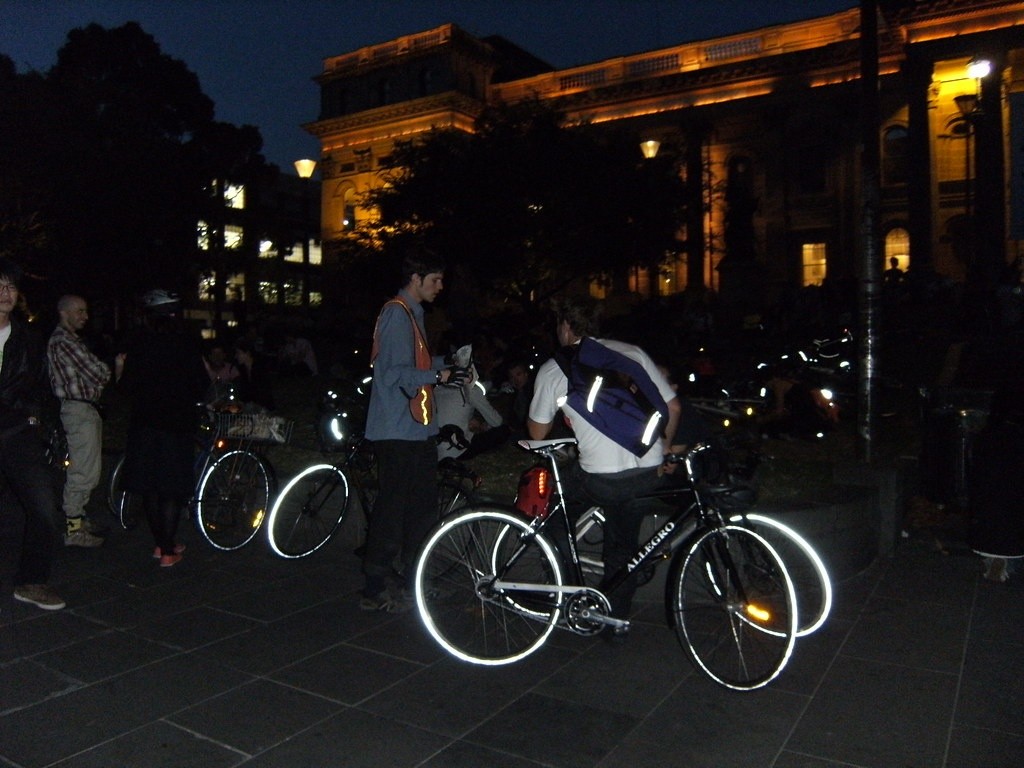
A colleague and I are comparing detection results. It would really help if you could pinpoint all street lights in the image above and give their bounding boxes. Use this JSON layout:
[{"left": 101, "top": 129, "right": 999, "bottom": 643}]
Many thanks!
[
  {"left": 294, "top": 158, "right": 317, "bottom": 304},
  {"left": 953, "top": 94, "right": 977, "bottom": 215},
  {"left": 640, "top": 140, "right": 660, "bottom": 297}
]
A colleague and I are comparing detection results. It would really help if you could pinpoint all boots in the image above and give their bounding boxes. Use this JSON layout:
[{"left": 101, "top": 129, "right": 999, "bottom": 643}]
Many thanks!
[
  {"left": 584, "top": 593, "right": 631, "bottom": 644},
  {"left": 513, "top": 562, "right": 579, "bottom": 624}
]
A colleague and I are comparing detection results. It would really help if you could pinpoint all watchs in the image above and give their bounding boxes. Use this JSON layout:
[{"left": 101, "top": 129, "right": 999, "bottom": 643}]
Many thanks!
[{"left": 436, "top": 370, "right": 442, "bottom": 384}]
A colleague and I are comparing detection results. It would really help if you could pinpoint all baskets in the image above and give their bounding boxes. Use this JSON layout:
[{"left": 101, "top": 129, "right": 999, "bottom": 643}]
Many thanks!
[{"left": 218, "top": 413, "right": 296, "bottom": 444}]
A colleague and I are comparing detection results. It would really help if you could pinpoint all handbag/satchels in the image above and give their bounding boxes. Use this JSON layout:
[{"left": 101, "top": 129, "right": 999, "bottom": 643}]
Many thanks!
[{"left": 555, "top": 335, "right": 670, "bottom": 458}]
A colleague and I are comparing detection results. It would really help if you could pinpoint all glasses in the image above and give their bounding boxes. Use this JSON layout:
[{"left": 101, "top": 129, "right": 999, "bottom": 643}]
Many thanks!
[{"left": 0, "top": 283, "right": 16, "bottom": 292}]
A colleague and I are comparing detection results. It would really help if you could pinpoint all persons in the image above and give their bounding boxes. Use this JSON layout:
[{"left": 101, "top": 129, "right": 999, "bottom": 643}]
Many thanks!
[
  {"left": 358, "top": 250, "right": 474, "bottom": 614},
  {"left": 0, "top": 258, "right": 318, "bottom": 611},
  {"left": 528, "top": 291, "right": 680, "bottom": 645},
  {"left": 883, "top": 257, "right": 903, "bottom": 281},
  {"left": 431, "top": 334, "right": 533, "bottom": 458},
  {"left": 656, "top": 342, "right": 803, "bottom": 506}
]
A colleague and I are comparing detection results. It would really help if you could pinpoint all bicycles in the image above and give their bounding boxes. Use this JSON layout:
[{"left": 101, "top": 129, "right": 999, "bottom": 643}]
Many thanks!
[
  {"left": 411, "top": 438, "right": 799, "bottom": 692},
  {"left": 106, "top": 402, "right": 278, "bottom": 553},
  {"left": 265, "top": 401, "right": 480, "bottom": 586},
  {"left": 489, "top": 441, "right": 835, "bottom": 640}
]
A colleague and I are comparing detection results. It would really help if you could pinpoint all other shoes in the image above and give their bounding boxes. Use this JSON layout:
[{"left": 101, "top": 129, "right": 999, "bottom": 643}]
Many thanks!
[{"left": 359, "top": 590, "right": 413, "bottom": 612}]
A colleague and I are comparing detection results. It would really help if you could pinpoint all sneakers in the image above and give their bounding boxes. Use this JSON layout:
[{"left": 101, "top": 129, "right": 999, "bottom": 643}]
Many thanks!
[
  {"left": 14, "top": 582, "right": 67, "bottom": 611},
  {"left": 62, "top": 528, "right": 104, "bottom": 546},
  {"left": 388, "top": 581, "right": 439, "bottom": 603},
  {"left": 160, "top": 553, "right": 183, "bottom": 567},
  {"left": 81, "top": 510, "right": 108, "bottom": 534},
  {"left": 153, "top": 543, "right": 186, "bottom": 559}
]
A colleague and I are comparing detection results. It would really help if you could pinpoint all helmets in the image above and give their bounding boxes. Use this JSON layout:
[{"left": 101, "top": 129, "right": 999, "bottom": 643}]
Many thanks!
[{"left": 144, "top": 288, "right": 183, "bottom": 306}]
